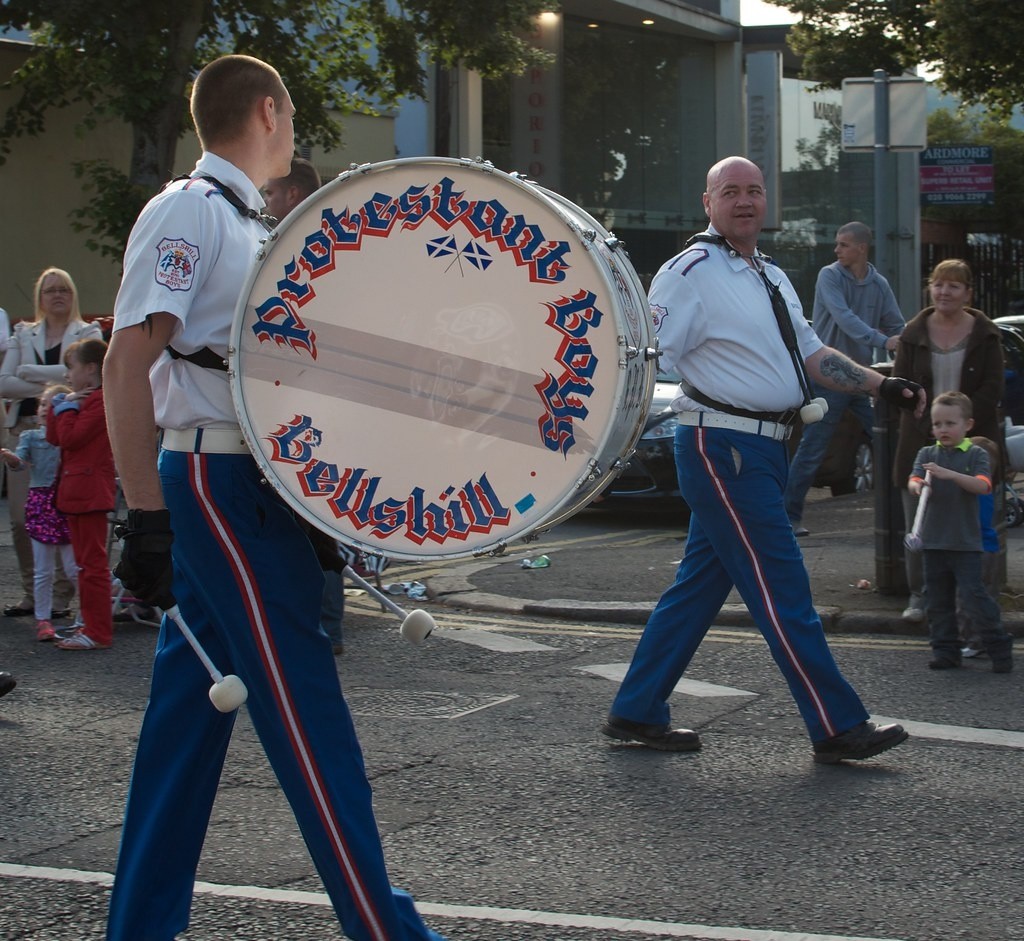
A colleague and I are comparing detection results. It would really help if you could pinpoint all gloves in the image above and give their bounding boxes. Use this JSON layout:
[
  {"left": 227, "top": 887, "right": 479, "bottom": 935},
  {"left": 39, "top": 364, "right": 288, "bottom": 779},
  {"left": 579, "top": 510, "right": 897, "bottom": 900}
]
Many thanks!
[{"left": 111, "top": 509, "right": 175, "bottom": 606}]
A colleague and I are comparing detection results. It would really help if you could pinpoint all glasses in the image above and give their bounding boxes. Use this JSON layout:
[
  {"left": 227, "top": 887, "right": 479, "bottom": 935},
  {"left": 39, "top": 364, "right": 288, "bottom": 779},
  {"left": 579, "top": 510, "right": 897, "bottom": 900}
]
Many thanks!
[{"left": 40, "top": 288, "right": 74, "bottom": 297}]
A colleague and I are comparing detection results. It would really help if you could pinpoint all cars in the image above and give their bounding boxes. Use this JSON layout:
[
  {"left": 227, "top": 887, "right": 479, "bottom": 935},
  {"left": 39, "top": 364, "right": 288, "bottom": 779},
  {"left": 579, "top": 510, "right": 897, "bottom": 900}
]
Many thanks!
[
  {"left": 609, "top": 371, "right": 682, "bottom": 495},
  {"left": 830, "top": 362, "right": 1024, "bottom": 496}
]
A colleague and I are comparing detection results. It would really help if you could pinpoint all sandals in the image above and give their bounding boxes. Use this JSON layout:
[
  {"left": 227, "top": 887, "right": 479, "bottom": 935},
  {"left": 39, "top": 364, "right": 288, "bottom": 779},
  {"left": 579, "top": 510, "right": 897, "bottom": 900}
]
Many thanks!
[{"left": 34, "top": 620, "right": 55, "bottom": 641}]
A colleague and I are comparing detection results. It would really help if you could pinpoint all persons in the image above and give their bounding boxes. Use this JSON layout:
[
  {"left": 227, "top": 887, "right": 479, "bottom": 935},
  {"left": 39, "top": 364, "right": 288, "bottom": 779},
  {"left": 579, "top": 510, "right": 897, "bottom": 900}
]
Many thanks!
[
  {"left": 890, "top": 257, "right": 1002, "bottom": 619},
  {"left": 1, "top": 386, "right": 79, "bottom": 639},
  {"left": 908, "top": 392, "right": 1014, "bottom": 674},
  {"left": 100, "top": 55, "right": 441, "bottom": 941},
  {"left": 785, "top": 222, "right": 906, "bottom": 537},
  {"left": 263, "top": 158, "right": 343, "bottom": 653},
  {"left": 0, "top": 267, "right": 154, "bottom": 620},
  {"left": 599, "top": 157, "right": 925, "bottom": 763},
  {"left": 46, "top": 340, "right": 115, "bottom": 650}
]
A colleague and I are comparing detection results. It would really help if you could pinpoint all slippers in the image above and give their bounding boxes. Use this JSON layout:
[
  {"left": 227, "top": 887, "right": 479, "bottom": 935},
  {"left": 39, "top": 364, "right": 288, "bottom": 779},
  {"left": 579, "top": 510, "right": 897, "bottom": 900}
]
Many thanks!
[{"left": 54, "top": 631, "right": 111, "bottom": 650}]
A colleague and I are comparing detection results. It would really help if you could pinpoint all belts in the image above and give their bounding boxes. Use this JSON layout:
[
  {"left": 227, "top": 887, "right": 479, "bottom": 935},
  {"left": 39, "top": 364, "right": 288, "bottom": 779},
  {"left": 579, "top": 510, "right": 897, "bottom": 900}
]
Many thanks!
[
  {"left": 161, "top": 428, "right": 253, "bottom": 456},
  {"left": 676, "top": 411, "right": 794, "bottom": 440}
]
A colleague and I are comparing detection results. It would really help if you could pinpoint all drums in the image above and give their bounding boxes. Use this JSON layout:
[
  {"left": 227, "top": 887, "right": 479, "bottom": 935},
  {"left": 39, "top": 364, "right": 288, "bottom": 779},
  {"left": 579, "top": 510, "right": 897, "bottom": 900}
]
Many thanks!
[{"left": 221, "top": 155, "right": 661, "bottom": 564}]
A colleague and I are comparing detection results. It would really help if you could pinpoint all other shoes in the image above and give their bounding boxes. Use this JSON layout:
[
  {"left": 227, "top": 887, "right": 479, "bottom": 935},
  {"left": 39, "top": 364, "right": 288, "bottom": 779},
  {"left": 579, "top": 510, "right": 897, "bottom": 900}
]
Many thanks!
[
  {"left": 113, "top": 608, "right": 157, "bottom": 623},
  {"left": 50, "top": 609, "right": 72, "bottom": 619},
  {"left": 991, "top": 656, "right": 1014, "bottom": 673},
  {"left": 2, "top": 602, "right": 35, "bottom": 617},
  {"left": 809, "top": 717, "right": 910, "bottom": 765},
  {"left": 790, "top": 518, "right": 811, "bottom": 536},
  {"left": 929, "top": 656, "right": 954, "bottom": 670},
  {"left": 901, "top": 607, "right": 924, "bottom": 625},
  {"left": 600, "top": 711, "right": 703, "bottom": 751},
  {"left": 0, "top": 671, "right": 17, "bottom": 697}
]
